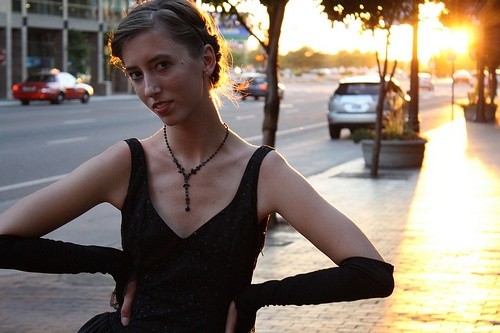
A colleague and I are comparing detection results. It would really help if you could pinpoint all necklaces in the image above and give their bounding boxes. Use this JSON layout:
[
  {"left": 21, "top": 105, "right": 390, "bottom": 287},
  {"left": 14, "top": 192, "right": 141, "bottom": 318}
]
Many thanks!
[{"left": 163, "top": 123, "right": 230, "bottom": 212}]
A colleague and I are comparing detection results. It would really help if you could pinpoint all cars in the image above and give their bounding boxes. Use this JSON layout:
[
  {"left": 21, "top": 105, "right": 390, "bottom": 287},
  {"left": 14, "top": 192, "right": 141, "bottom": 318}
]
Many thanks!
[
  {"left": 327, "top": 76, "right": 405, "bottom": 140},
  {"left": 241, "top": 75, "right": 286, "bottom": 100},
  {"left": 419, "top": 73, "right": 434, "bottom": 91},
  {"left": 11, "top": 69, "right": 94, "bottom": 107},
  {"left": 453, "top": 70, "right": 471, "bottom": 83}
]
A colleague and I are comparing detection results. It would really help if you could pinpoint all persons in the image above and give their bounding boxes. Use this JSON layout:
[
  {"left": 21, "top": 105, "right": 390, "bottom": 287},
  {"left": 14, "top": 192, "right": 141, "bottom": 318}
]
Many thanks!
[{"left": 0, "top": 1, "right": 394, "bottom": 333}]
